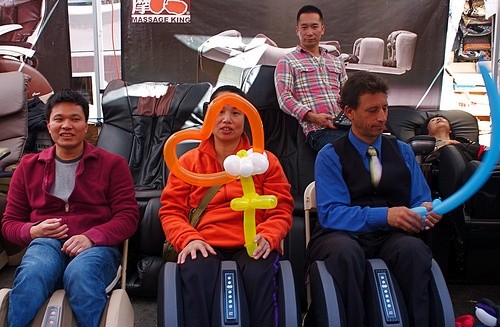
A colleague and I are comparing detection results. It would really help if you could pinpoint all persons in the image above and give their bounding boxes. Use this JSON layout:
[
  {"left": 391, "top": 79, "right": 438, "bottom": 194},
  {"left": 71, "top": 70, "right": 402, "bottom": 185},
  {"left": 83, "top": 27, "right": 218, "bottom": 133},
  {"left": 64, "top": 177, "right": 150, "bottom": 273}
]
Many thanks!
[
  {"left": 158, "top": 84, "right": 294, "bottom": 327},
  {"left": 1, "top": 90, "right": 138, "bottom": 327},
  {"left": 315, "top": 70, "right": 444, "bottom": 327},
  {"left": 274, "top": 5, "right": 352, "bottom": 153},
  {"left": 421, "top": 114, "right": 488, "bottom": 225}
]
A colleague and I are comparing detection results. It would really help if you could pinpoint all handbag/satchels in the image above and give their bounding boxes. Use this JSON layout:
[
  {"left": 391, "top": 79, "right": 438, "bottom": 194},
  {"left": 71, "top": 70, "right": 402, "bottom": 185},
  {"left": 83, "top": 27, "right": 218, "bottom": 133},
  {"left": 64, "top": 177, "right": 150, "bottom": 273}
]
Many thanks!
[{"left": 163, "top": 240, "right": 178, "bottom": 262}]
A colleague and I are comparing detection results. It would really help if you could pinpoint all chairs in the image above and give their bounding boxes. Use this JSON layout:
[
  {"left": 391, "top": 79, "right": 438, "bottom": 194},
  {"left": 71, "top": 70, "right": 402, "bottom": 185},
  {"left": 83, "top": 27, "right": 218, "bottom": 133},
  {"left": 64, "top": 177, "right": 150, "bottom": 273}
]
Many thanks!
[
  {"left": 156, "top": 240, "right": 298, "bottom": 327},
  {"left": 238, "top": 65, "right": 316, "bottom": 197},
  {"left": 0, "top": 236, "right": 136, "bottom": 327},
  {"left": 95, "top": 79, "right": 213, "bottom": 305},
  {"left": 296, "top": 139, "right": 455, "bottom": 327},
  {"left": 386, "top": 105, "right": 500, "bottom": 286}
]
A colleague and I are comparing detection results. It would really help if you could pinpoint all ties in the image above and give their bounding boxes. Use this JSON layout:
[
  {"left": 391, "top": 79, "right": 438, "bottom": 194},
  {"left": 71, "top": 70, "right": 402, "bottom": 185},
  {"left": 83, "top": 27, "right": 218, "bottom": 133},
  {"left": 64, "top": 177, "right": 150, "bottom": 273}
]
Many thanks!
[{"left": 367, "top": 145, "right": 382, "bottom": 186}]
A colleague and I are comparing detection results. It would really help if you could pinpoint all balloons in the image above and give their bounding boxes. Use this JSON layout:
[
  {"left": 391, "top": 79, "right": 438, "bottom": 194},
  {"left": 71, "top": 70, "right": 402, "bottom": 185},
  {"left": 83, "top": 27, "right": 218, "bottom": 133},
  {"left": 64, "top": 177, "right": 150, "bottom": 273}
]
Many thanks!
[
  {"left": 410, "top": 62, "right": 500, "bottom": 227},
  {"left": 164, "top": 91, "right": 277, "bottom": 257}
]
